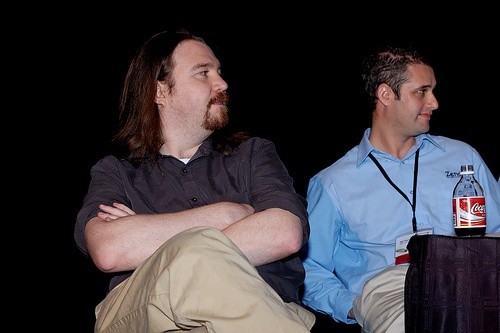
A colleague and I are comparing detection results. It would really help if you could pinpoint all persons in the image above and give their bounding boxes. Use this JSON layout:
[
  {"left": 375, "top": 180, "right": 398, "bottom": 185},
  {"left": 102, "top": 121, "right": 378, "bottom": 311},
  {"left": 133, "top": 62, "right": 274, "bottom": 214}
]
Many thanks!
[
  {"left": 302, "top": 44, "right": 500, "bottom": 333},
  {"left": 74, "top": 30, "right": 316, "bottom": 333}
]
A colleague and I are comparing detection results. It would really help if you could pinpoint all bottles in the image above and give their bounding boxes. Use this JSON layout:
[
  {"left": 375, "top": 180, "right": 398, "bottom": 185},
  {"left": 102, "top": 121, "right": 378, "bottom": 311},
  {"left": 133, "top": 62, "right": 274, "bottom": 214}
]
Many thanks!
[{"left": 452, "top": 165, "right": 486, "bottom": 237}]
